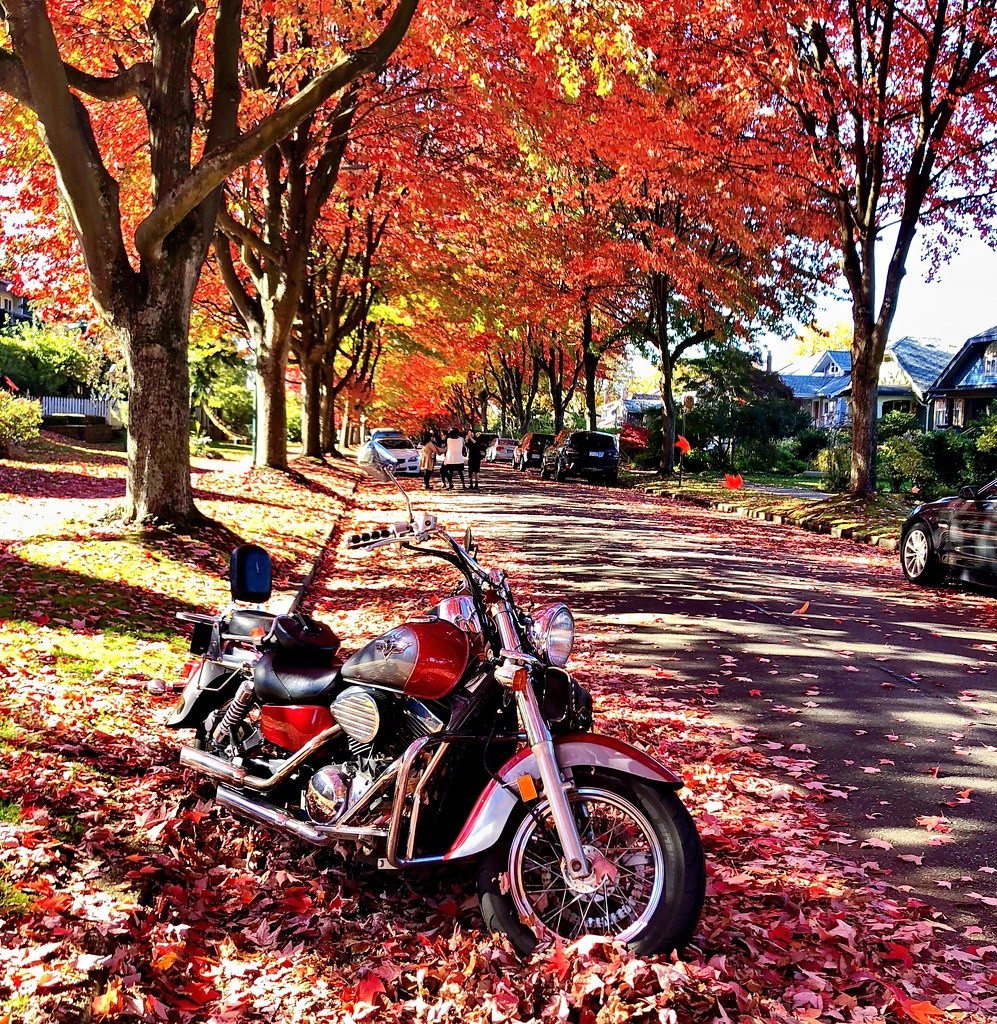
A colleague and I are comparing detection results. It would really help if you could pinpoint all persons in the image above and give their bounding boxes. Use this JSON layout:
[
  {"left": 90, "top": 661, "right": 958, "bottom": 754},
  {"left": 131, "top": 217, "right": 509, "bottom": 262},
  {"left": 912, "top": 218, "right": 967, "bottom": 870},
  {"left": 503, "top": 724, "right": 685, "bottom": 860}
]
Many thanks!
[{"left": 419, "top": 427, "right": 481, "bottom": 491}]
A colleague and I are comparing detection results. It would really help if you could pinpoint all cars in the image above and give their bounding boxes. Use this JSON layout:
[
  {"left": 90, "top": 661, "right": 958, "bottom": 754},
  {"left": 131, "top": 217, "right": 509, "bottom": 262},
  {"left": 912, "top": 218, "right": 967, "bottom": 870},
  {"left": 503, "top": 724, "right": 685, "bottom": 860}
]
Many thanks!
[
  {"left": 368, "top": 427, "right": 421, "bottom": 474},
  {"left": 899, "top": 479, "right": 997, "bottom": 590},
  {"left": 476, "top": 430, "right": 620, "bottom": 485}
]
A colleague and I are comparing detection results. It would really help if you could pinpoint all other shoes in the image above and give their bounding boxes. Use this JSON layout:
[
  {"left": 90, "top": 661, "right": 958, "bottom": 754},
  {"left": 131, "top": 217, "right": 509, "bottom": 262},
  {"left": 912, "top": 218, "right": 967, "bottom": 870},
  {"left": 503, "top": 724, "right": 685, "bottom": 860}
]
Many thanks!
[
  {"left": 468, "top": 484, "right": 473, "bottom": 490},
  {"left": 463, "top": 485, "right": 467, "bottom": 490},
  {"left": 449, "top": 484, "right": 454, "bottom": 489},
  {"left": 425, "top": 484, "right": 433, "bottom": 489},
  {"left": 442, "top": 483, "right": 446, "bottom": 488},
  {"left": 474, "top": 484, "right": 478, "bottom": 490}
]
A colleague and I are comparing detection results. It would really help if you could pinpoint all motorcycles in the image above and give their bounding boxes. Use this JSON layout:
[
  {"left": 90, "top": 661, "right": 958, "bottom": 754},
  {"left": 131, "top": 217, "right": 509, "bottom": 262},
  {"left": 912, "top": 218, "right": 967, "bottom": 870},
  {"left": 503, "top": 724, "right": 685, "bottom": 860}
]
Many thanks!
[{"left": 147, "top": 441, "right": 706, "bottom": 964}]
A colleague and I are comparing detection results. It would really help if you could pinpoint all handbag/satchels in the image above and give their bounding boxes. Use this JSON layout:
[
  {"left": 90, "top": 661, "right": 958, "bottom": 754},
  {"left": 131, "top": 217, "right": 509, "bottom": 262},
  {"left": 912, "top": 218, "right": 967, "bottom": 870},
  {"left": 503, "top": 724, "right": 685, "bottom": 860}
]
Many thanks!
[{"left": 462, "top": 437, "right": 467, "bottom": 457}]
